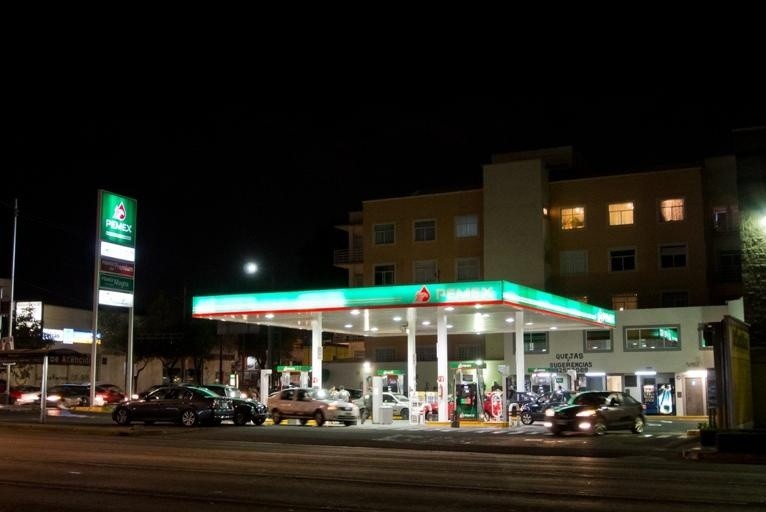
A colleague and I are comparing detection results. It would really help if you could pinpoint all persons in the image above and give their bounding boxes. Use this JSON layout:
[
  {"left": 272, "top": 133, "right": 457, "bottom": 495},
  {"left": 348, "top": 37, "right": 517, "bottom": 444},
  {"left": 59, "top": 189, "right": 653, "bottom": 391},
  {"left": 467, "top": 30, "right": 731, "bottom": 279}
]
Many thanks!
[
  {"left": 328, "top": 384, "right": 339, "bottom": 400},
  {"left": 339, "top": 385, "right": 350, "bottom": 402}
]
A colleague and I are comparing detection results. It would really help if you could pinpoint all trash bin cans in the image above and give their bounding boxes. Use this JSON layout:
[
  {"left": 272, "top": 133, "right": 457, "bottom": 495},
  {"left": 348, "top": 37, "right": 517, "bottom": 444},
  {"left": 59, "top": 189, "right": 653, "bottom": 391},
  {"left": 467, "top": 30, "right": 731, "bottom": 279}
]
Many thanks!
[{"left": 379, "top": 406, "right": 393, "bottom": 425}]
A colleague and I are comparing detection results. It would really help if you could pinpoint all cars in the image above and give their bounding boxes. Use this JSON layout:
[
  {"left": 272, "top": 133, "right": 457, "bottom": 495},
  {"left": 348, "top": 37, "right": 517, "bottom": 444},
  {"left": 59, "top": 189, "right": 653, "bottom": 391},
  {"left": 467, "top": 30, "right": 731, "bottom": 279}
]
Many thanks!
[{"left": 544, "top": 390, "right": 647, "bottom": 441}]
